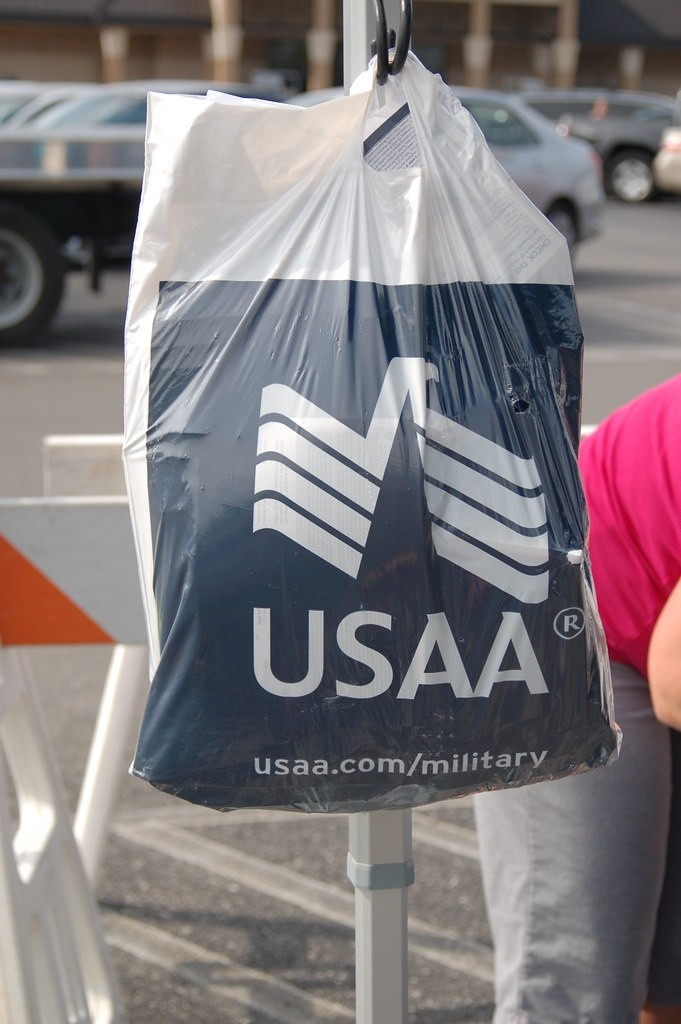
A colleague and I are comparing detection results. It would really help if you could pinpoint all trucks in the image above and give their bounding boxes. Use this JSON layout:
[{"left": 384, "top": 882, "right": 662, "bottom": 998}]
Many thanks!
[{"left": 0, "top": 136, "right": 179, "bottom": 350}]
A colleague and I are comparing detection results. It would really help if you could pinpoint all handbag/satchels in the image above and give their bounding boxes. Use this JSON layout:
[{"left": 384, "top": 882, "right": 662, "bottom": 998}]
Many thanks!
[{"left": 122, "top": 47, "right": 624, "bottom": 816}]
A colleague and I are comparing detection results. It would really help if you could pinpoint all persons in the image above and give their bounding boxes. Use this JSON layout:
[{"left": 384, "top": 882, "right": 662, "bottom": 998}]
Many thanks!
[{"left": 471, "top": 374, "right": 681, "bottom": 1024}]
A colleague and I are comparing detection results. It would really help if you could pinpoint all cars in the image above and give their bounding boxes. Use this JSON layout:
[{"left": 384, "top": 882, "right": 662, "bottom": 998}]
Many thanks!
[
  {"left": 520, "top": 91, "right": 681, "bottom": 205},
  {"left": 2, "top": 78, "right": 291, "bottom": 134},
  {"left": 293, "top": 83, "right": 603, "bottom": 271}
]
